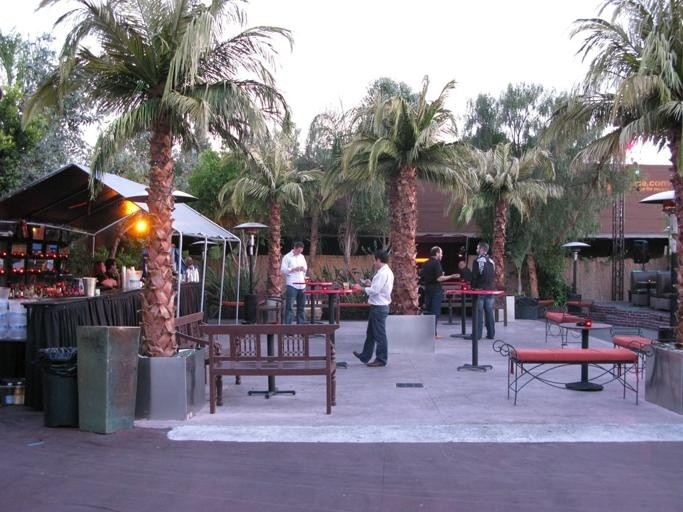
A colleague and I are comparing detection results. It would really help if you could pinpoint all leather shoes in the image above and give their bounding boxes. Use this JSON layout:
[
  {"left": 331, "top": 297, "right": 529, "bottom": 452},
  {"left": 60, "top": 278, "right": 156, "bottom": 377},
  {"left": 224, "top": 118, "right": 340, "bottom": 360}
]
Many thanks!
[
  {"left": 352, "top": 351, "right": 386, "bottom": 368},
  {"left": 463, "top": 332, "right": 496, "bottom": 341}
]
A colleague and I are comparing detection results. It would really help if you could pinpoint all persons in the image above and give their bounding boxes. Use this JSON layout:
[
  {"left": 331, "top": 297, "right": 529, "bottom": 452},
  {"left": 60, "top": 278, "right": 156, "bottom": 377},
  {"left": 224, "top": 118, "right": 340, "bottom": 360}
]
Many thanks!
[
  {"left": 93, "top": 240, "right": 196, "bottom": 292},
  {"left": 280, "top": 240, "right": 308, "bottom": 338},
  {"left": 458, "top": 242, "right": 496, "bottom": 339},
  {"left": 418, "top": 246, "right": 461, "bottom": 339},
  {"left": 352, "top": 249, "right": 394, "bottom": 366}
]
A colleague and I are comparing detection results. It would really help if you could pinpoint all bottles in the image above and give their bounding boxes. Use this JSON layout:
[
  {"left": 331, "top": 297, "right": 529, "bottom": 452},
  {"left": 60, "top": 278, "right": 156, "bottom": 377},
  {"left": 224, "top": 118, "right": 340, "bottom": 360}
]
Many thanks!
[
  {"left": 4, "top": 382, "right": 13, "bottom": 405},
  {"left": 186, "top": 268, "right": 199, "bottom": 282},
  {"left": 13, "top": 381, "right": 22, "bottom": 405},
  {"left": 8, "top": 283, "right": 70, "bottom": 298}
]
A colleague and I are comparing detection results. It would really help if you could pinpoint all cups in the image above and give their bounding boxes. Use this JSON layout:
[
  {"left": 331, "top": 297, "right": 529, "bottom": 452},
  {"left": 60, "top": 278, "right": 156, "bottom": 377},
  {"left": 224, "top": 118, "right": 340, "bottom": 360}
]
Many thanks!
[
  {"left": 583, "top": 316, "right": 592, "bottom": 326},
  {"left": 81, "top": 277, "right": 97, "bottom": 297},
  {"left": 351, "top": 284, "right": 356, "bottom": 298},
  {"left": 0, "top": 287, "right": 10, "bottom": 299},
  {"left": 342, "top": 282, "right": 348, "bottom": 291},
  {"left": 74, "top": 278, "right": 84, "bottom": 296},
  {"left": 304, "top": 277, "right": 309, "bottom": 283},
  {"left": 120, "top": 264, "right": 137, "bottom": 287},
  {"left": 460, "top": 284, "right": 468, "bottom": 291},
  {"left": 94, "top": 289, "right": 99, "bottom": 296}
]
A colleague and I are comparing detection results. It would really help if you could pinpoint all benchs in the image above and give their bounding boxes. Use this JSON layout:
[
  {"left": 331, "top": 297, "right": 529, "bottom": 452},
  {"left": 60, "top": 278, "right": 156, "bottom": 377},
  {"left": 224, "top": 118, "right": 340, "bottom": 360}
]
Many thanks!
[
  {"left": 491, "top": 339, "right": 645, "bottom": 407},
  {"left": 609, "top": 327, "right": 663, "bottom": 378},
  {"left": 196, "top": 322, "right": 341, "bottom": 417},
  {"left": 539, "top": 300, "right": 591, "bottom": 347},
  {"left": 323, "top": 292, "right": 385, "bottom": 322},
  {"left": 174, "top": 312, "right": 222, "bottom": 384}
]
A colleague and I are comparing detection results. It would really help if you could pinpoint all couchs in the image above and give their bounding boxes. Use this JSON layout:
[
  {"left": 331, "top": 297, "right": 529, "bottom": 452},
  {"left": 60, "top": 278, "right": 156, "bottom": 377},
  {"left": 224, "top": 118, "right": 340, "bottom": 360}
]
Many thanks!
[{"left": 624, "top": 267, "right": 673, "bottom": 310}]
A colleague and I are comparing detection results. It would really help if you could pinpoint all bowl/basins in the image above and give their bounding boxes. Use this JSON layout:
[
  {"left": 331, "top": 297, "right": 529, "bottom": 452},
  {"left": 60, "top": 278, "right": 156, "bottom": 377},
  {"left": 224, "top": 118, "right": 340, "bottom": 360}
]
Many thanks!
[{"left": 38, "top": 347, "right": 78, "bottom": 363}]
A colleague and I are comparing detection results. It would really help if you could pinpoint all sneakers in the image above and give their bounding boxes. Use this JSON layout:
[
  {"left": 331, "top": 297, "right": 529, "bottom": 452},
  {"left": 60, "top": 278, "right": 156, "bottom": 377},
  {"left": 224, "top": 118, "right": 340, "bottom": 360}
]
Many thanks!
[{"left": 435, "top": 335, "right": 444, "bottom": 340}]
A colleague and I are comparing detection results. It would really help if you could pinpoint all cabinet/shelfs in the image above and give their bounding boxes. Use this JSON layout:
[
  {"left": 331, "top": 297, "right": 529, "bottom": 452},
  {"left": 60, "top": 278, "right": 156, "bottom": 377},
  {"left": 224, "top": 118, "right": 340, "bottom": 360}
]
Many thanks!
[{"left": 0, "top": 221, "right": 71, "bottom": 295}]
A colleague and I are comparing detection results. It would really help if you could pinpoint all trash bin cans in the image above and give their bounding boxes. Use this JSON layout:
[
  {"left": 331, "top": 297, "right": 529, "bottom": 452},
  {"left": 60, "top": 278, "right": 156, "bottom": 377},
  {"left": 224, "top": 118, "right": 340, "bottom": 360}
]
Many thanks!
[
  {"left": 39, "top": 347, "right": 79, "bottom": 428},
  {"left": 518, "top": 297, "right": 539, "bottom": 320}
]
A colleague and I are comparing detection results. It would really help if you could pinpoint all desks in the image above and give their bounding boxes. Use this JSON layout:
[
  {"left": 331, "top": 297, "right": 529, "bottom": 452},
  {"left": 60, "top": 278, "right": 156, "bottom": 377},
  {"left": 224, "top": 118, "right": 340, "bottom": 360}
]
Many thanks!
[
  {"left": 291, "top": 281, "right": 331, "bottom": 328},
  {"left": 445, "top": 288, "right": 509, "bottom": 372},
  {"left": 438, "top": 279, "right": 469, "bottom": 326},
  {"left": 304, "top": 288, "right": 366, "bottom": 370},
  {"left": 557, "top": 320, "right": 615, "bottom": 392}
]
